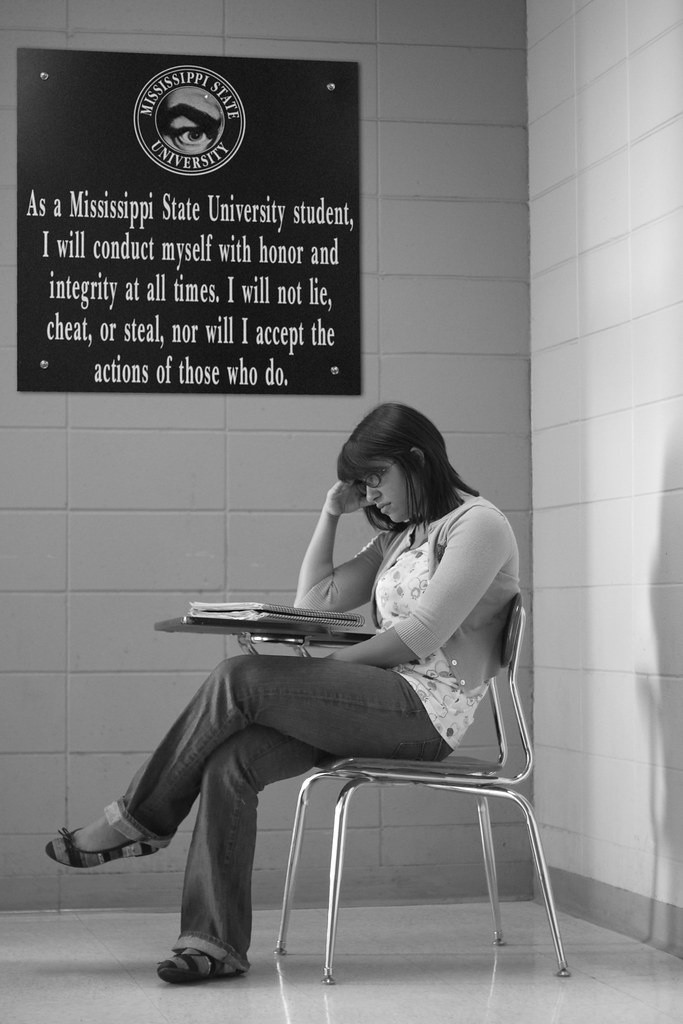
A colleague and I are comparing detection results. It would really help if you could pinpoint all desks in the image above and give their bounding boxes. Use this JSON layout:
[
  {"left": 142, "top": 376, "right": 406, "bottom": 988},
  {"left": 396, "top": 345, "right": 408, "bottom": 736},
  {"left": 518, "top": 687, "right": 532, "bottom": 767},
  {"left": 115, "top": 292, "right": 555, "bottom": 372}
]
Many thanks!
[{"left": 152, "top": 616, "right": 378, "bottom": 657}]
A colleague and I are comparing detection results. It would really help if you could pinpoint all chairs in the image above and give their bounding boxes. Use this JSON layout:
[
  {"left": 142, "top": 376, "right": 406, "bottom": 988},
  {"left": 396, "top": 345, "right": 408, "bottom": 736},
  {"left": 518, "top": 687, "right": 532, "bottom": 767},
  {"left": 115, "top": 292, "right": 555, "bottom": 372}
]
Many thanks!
[{"left": 276, "top": 591, "right": 574, "bottom": 985}]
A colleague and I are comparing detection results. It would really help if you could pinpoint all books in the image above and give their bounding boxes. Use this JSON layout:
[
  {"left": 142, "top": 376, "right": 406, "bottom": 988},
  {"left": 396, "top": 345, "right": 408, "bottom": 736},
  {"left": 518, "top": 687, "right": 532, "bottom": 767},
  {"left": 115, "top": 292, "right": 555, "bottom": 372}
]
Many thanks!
[{"left": 190, "top": 601, "right": 366, "bottom": 629}]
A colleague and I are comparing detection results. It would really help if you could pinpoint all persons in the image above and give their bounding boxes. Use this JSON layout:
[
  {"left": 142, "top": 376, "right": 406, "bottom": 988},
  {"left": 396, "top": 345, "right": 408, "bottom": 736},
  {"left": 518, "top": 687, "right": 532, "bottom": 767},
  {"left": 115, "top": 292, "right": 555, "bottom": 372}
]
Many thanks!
[{"left": 46, "top": 402, "right": 519, "bottom": 982}]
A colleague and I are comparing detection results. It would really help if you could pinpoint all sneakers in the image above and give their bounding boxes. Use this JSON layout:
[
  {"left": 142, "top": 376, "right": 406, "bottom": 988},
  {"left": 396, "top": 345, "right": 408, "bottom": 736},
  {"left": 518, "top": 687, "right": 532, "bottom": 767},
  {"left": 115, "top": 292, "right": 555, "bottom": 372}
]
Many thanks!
[
  {"left": 156, "top": 947, "right": 244, "bottom": 985},
  {"left": 45, "top": 827, "right": 158, "bottom": 869}
]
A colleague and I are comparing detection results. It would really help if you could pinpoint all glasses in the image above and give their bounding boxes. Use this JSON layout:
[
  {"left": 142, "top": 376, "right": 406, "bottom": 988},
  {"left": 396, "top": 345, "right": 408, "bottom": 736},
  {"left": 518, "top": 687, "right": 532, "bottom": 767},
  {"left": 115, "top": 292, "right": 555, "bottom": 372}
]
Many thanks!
[{"left": 355, "top": 461, "right": 398, "bottom": 496}]
